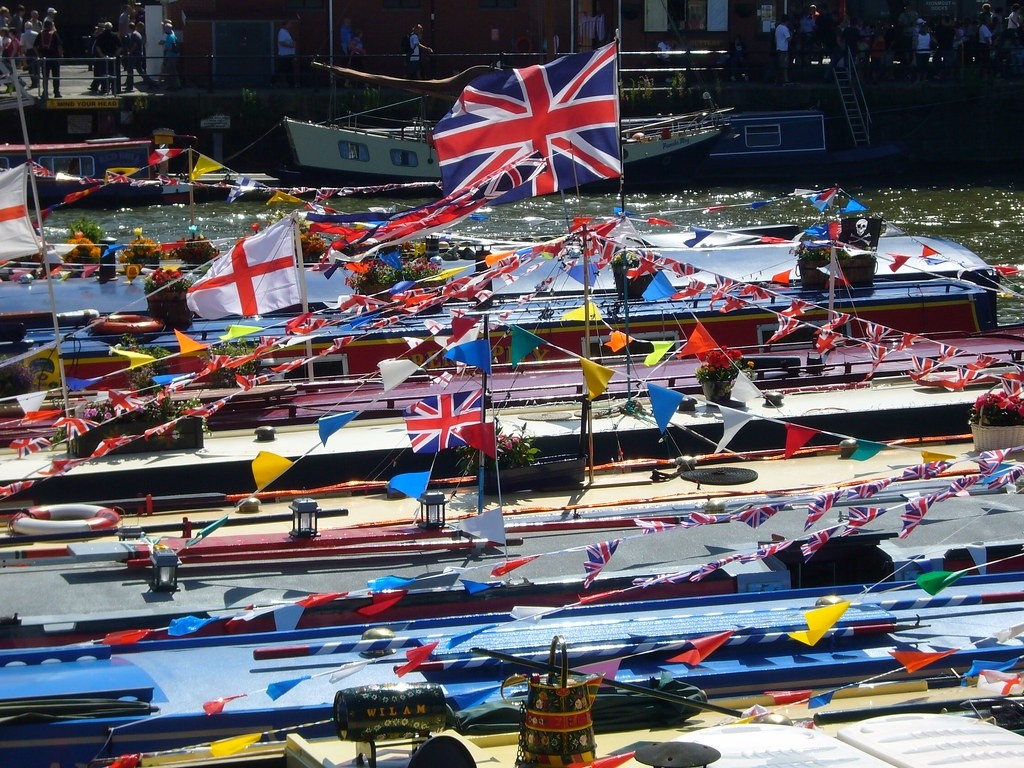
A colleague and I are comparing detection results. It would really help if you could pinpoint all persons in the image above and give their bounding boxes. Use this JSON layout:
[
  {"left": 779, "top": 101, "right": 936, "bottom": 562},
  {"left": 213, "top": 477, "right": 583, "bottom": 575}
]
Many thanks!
[
  {"left": 34, "top": 21, "right": 61, "bottom": 99},
  {"left": 798, "top": 1, "right": 1021, "bottom": 82},
  {"left": 656, "top": 33, "right": 749, "bottom": 81},
  {"left": 408, "top": 21, "right": 433, "bottom": 80},
  {"left": 96, "top": 21, "right": 123, "bottom": 94},
  {"left": 340, "top": 18, "right": 353, "bottom": 54},
  {"left": 344, "top": 26, "right": 369, "bottom": 88},
  {"left": 276, "top": 22, "right": 298, "bottom": 92},
  {"left": 88, "top": 1, "right": 153, "bottom": 95},
  {"left": 159, "top": 18, "right": 183, "bottom": 90},
  {"left": 775, "top": 14, "right": 795, "bottom": 83},
  {"left": 124, "top": 22, "right": 152, "bottom": 90},
  {"left": 0, "top": 4, "right": 61, "bottom": 97}
]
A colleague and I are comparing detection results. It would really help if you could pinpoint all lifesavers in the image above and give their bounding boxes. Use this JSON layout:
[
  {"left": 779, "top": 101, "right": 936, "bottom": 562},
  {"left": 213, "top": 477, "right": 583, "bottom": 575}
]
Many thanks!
[
  {"left": 90, "top": 312, "right": 161, "bottom": 333},
  {"left": 11, "top": 502, "right": 117, "bottom": 536}
]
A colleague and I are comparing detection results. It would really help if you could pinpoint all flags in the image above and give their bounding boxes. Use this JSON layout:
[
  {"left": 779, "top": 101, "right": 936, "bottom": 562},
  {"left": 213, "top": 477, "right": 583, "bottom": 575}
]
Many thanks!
[
  {"left": 584, "top": 448, "right": 1024, "bottom": 625},
  {"left": 405, "top": 387, "right": 481, "bottom": 457},
  {"left": 1, "top": 243, "right": 571, "bottom": 502},
  {"left": 0, "top": 161, "right": 46, "bottom": 268},
  {"left": 579, "top": 227, "right": 1024, "bottom": 409},
  {"left": 29, "top": 162, "right": 435, "bottom": 206},
  {"left": 433, "top": 37, "right": 624, "bottom": 207},
  {"left": 977, "top": 668, "right": 1021, "bottom": 695},
  {"left": 189, "top": 210, "right": 301, "bottom": 322}
]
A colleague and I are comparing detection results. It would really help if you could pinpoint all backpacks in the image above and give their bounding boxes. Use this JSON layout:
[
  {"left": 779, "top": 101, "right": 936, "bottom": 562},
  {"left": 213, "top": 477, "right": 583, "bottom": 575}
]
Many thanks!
[{"left": 401, "top": 30, "right": 419, "bottom": 54}]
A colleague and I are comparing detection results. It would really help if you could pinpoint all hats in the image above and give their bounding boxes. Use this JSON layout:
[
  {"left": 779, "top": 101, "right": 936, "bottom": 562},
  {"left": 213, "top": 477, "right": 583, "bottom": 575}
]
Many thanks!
[
  {"left": 95, "top": 23, "right": 105, "bottom": 28},
  {"left": 161, "top": 19, "right": 172, "bottom": 26},
  {"left": 916, "top": 18, "right": 926, "bottom": 24},
  {"left": 48, "top": 8, "right": 57, "bottom": 14}
]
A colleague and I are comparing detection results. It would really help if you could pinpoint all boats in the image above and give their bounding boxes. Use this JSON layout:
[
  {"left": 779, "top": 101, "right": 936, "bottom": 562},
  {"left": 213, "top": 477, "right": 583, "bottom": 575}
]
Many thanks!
[
  {"left": 280, "top": 59, "right": 736, "bottom": 195},
  {"left": 0, "top": 58, "right": 1024, "bottom": 768}
]
